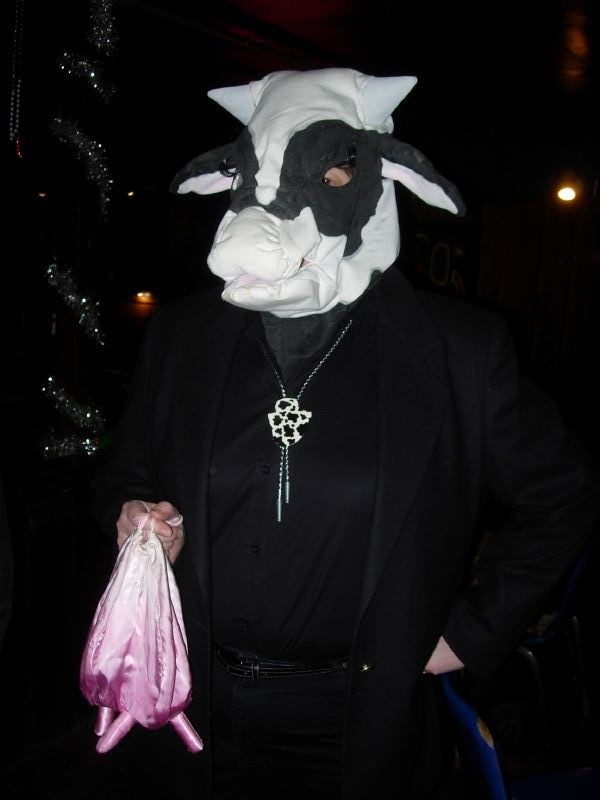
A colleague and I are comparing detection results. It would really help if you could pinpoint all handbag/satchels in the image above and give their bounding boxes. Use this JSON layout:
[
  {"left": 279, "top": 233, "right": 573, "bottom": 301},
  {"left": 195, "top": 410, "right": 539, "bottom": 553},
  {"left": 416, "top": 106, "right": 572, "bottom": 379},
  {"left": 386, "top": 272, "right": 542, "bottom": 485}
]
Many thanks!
[{"left": 80, "top": 515, "right": 204, "bottom": 753}]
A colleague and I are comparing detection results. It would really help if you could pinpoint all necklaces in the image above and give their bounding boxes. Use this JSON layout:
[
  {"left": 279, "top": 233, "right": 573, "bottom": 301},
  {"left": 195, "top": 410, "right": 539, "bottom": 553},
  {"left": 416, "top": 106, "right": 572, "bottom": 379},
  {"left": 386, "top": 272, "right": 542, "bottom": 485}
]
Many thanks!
[{"left": 254, "top": 320, "right": 353, "bottom": 522}]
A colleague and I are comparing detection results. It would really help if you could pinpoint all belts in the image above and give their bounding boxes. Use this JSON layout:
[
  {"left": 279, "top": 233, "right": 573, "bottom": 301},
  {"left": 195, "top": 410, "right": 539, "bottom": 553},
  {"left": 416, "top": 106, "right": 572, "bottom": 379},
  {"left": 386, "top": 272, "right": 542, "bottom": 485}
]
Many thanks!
[{"left": 211, "top": 642, "right": 350, "bottom": 679}]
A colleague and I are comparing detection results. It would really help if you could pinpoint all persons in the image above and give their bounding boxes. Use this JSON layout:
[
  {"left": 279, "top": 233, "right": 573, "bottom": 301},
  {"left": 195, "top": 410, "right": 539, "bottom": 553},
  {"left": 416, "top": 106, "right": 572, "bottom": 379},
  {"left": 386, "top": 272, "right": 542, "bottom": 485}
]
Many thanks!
[{"left": 86, "top": 66, "right": 580, "bottom": 800}]
